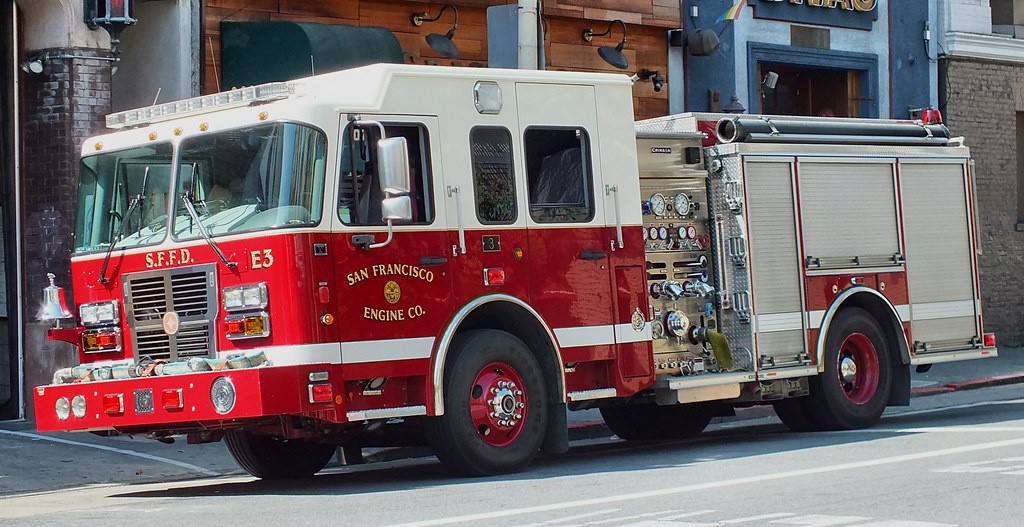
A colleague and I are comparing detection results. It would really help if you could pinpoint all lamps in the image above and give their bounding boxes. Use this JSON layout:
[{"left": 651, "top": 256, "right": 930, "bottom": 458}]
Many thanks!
[
  {"left": 20, "top": 50, "right": 47, "bottom": 73},
  {"left": 722, "top": 93, "right": 746, "bottom": 113},
  {"left": 761, "top": 71, "right": 778, "bottom": 89},
  {"left": 412, "top": 4, "right": 459, "bottom": 58},
  {"left": 687, "top": 28, "right": 720, "bottom": 56},
  {"left": 81, "top": 0, "right": 138, "bottom": 61},
  {"left": 641, "top": 68, "right": 664, "bottom": 92},
  {"left": 583, "top": 19, "right": 628, "bottom": 69}
]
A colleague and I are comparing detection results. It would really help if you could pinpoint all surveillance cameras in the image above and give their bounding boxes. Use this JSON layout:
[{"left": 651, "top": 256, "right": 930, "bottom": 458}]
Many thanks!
[{"left": 652, "top": 76, "right": 664, "bottom": 92}]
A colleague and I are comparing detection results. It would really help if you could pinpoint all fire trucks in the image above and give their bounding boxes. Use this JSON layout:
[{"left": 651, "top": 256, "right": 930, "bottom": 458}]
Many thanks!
[{"left": 33, "top": 64, "right": 1000, "bottom": 490}]
